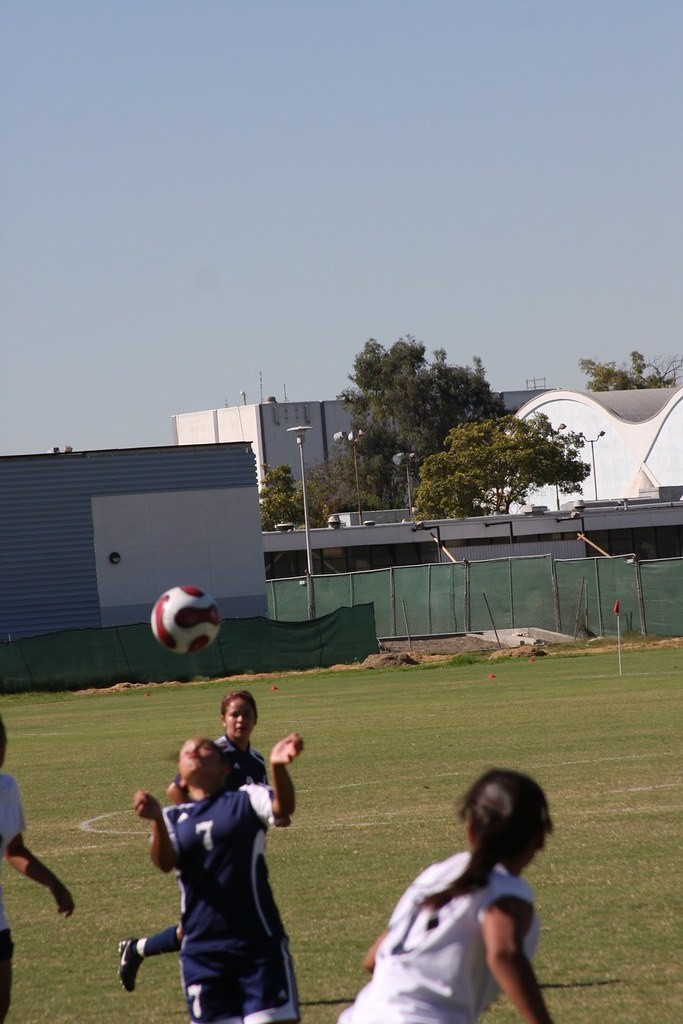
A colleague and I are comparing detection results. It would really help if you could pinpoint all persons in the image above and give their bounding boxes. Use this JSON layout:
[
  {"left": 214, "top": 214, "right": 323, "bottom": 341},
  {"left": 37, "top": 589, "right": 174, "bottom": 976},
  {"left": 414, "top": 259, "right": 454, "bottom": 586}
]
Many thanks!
[
  {"left": 0, "top": 720, "right": 74, "bottom": 1024},
  {"left": 338, "top": 769, "right": 556, "bottom": 1024},
  {"left": 133, "top": 734, "right": 303, "bottom": 1024},
  {"left": 119, "top": 691, "right": 269, "bottom": 991}
]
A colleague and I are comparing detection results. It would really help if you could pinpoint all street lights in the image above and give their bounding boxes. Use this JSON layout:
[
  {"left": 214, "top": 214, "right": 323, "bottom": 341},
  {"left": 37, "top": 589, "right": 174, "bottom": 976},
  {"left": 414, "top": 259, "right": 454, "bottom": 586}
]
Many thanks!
[
  {"left": 393, "top": 452, "right": 416, "bottom": 522},
  {"left": 287, "top": 425, "right": 316, "bottom": 619},
  {"left": 334, "top": 428, "right": 367, "bottom": 526},
  {"left": 578, "top": 431, "right": 605, "bottom": 500},
  {"left": 546, "top": 423, "right": 566, "bottom": 510}
]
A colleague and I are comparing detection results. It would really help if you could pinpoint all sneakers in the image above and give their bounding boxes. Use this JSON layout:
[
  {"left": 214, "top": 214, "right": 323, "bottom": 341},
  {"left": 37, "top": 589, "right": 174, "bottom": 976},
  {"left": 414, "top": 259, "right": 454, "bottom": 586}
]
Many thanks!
[{"left": 118, "top": 937, "right": 144, "bottom": 993}]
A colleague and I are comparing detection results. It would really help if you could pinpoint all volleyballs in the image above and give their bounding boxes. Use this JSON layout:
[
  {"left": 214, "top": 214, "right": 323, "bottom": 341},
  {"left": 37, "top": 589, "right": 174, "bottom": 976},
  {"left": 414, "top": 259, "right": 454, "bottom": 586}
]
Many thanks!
[{"left": 149, "top": 584, "right": 223, "bottom": 656}]
